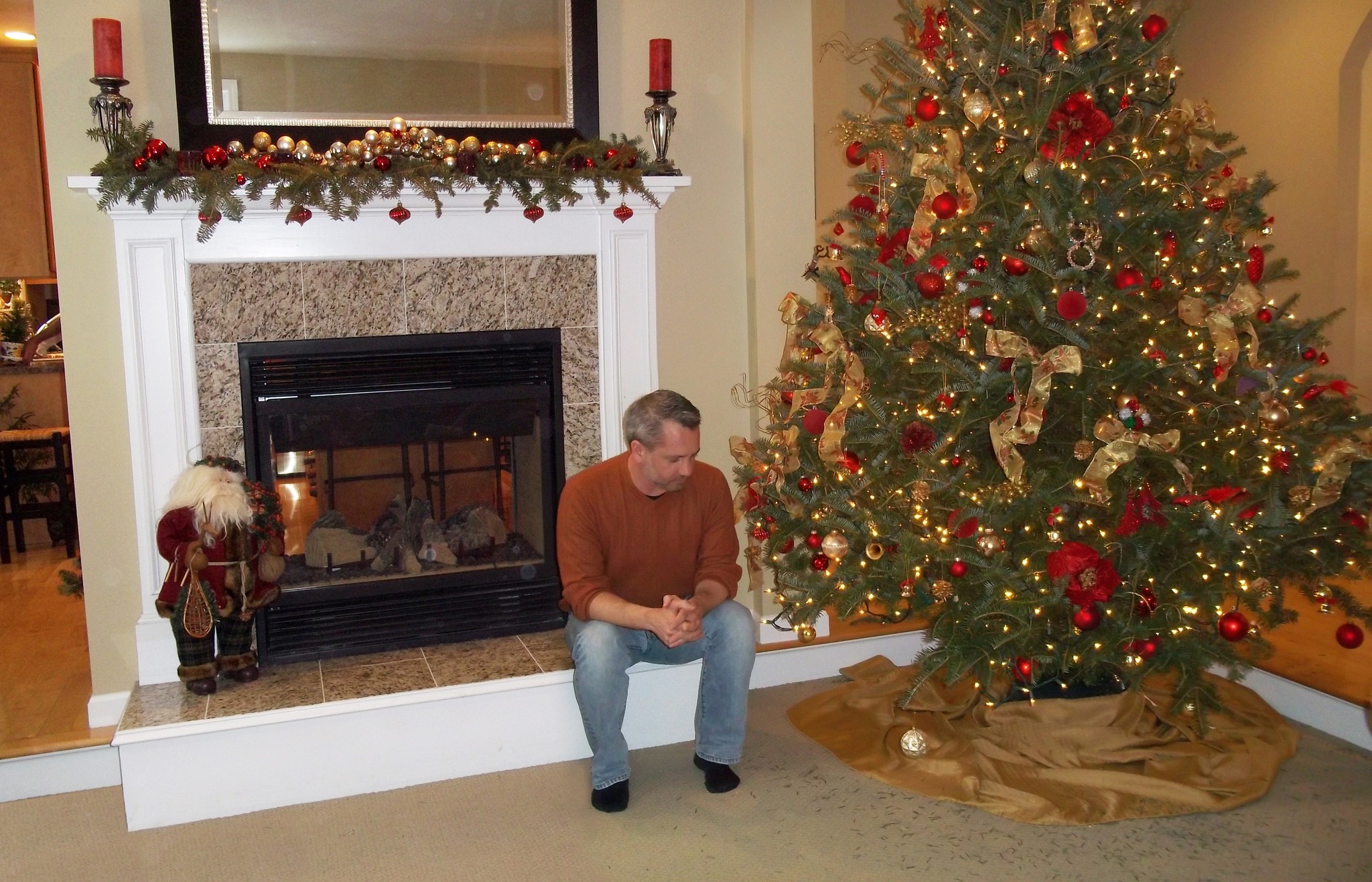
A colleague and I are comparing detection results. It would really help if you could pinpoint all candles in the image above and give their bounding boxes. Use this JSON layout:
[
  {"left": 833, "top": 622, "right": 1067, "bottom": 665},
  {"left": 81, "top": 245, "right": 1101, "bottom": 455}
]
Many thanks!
[
  {"left": 648, "top": 34, "right": 673, "bottom": 92},
  {"left": 93, "top": 19, "right": 122, "bottom": 80}
]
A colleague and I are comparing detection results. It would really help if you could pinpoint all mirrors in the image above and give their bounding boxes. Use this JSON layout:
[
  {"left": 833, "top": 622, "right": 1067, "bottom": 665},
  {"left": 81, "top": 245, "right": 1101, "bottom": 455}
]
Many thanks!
[{"left": 168, "top": 2, "right": 577, "bottom": 142}]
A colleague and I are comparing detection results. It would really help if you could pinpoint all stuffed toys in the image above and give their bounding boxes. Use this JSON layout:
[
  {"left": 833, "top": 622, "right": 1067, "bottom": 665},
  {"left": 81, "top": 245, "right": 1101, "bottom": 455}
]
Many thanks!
[{"left": 156, "top": 455, "right": 288, "bottom": 695}]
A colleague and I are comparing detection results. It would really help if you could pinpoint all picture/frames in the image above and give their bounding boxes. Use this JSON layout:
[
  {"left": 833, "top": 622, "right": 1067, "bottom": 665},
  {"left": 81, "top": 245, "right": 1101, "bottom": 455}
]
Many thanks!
[{"left": 17, "top": 275, "right": 64, "bottom": 369}]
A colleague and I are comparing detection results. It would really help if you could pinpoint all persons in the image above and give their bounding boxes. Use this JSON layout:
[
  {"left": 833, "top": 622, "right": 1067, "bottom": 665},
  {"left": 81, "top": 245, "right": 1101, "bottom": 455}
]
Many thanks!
[
  {"left": 20, "top": 312, "right": 61, "bottom": 363},
  {"left": 557, "top": 389, "right": 755, "bottom": 812}
]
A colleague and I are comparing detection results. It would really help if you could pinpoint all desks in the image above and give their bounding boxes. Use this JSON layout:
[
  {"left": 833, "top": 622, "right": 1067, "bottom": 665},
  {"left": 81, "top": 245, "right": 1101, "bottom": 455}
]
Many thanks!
[{"left": 1, "top": 424, "right": 81, "bottom": 565}]
